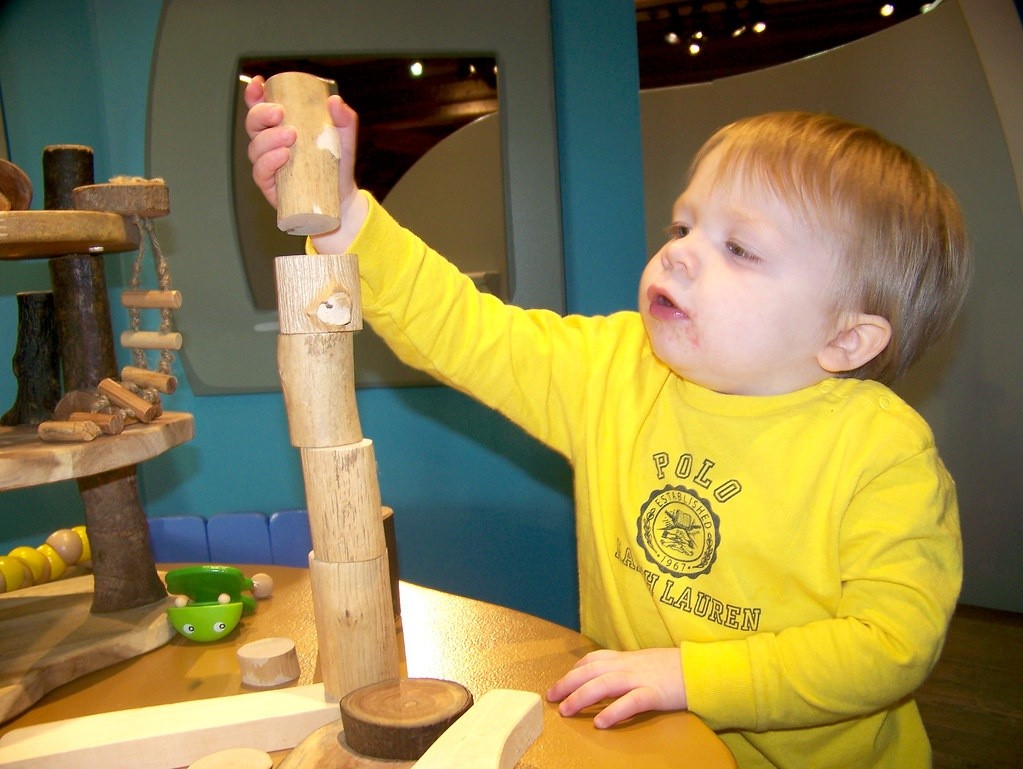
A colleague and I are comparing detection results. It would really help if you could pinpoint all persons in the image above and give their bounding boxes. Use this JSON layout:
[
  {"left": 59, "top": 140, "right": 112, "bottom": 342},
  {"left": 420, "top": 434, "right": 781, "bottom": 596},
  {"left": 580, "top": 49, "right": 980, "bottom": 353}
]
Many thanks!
[{"left": 245, "top": 73, "right": 963, "bottom": 769}]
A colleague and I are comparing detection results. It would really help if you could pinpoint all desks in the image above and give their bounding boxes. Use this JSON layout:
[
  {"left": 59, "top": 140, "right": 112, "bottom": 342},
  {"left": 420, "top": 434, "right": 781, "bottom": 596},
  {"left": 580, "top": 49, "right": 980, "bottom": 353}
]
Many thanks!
[{"left": 0, "top": 562, "right": 740, "bottom": 769}]
